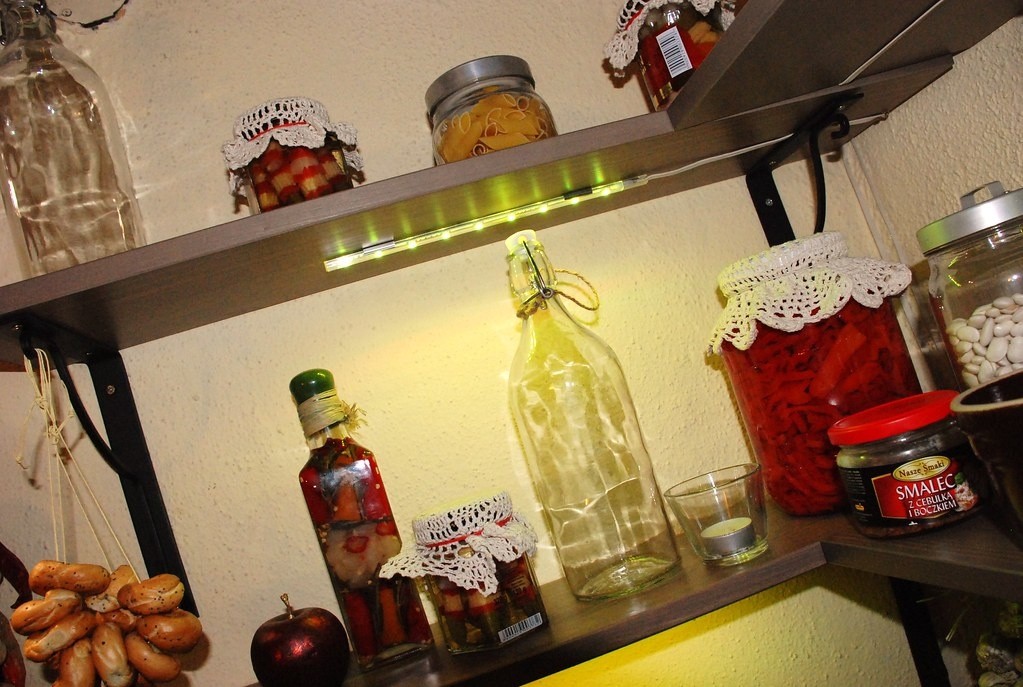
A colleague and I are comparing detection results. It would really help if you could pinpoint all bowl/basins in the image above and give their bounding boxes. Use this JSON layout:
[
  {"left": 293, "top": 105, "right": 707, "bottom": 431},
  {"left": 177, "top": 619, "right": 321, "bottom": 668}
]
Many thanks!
[{"left": 950, "top": 367, "right": 1023, "bottom": 536}]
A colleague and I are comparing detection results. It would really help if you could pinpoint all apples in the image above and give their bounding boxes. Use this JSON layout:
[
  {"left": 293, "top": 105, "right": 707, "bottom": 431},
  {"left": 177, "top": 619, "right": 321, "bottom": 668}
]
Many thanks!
[{"left": 250, "top": 592, "right": 350, "bottom": 687}]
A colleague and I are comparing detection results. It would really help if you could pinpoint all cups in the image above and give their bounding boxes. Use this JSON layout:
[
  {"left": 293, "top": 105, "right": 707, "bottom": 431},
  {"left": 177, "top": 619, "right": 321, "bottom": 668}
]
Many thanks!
[{"left": 663, "top": 462, "right": 765, "bottom": 569}]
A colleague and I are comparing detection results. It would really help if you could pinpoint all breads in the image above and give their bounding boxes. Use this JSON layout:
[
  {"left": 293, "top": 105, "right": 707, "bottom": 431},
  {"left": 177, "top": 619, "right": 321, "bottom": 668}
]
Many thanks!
[{"left": 11, "top": 559, "right": 203, "bottom": 687}]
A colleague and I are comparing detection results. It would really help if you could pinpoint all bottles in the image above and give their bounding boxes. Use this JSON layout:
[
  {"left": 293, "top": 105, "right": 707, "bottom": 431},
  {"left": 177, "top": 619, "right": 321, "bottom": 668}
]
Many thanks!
[
  {"left": 0, "top": 0, "right": 146, "bottom": 278},
  {"left": 504, "top": 230, "right": 680, "bottom": 602},
  {"left": 290, "top": 368, "right": 434, "bottom": 671}
]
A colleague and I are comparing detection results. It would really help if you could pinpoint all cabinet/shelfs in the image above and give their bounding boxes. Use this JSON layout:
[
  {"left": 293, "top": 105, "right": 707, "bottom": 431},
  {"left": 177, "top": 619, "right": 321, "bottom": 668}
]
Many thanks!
[{"left": 0, "top": 0, "right": 1023, "bottom": 687}]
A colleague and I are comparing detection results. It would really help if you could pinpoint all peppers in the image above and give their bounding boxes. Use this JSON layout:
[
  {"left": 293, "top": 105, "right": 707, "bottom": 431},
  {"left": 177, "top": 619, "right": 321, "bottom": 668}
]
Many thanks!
[{"left": 301, "top": 448, "right": 432, "bottom": 665}]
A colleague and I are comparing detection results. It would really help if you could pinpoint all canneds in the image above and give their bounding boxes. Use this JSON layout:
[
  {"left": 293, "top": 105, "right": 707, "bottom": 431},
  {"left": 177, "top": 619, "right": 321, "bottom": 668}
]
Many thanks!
[{"left": 828, "top": 390, "right": 988, "bottom": 538}]
